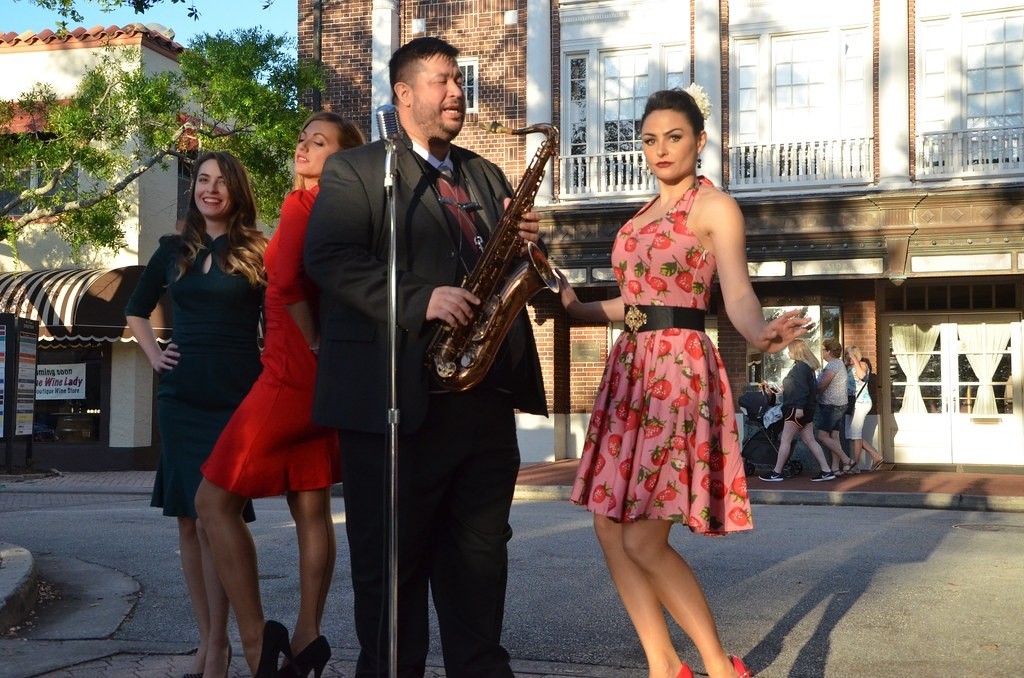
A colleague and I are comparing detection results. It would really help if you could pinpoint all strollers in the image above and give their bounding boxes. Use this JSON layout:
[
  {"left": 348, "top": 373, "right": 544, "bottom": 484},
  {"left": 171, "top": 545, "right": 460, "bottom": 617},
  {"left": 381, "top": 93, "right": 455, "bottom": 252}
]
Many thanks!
[{"left": 737, "top": 382, "right": 803, "bottom": 478}]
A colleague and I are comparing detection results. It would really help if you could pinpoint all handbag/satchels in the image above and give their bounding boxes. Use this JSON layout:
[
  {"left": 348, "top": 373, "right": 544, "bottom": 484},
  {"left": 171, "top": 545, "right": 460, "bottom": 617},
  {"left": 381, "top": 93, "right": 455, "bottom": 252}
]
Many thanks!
[{"left": 844, "top": 396, "right": 856, "bottom": 415}]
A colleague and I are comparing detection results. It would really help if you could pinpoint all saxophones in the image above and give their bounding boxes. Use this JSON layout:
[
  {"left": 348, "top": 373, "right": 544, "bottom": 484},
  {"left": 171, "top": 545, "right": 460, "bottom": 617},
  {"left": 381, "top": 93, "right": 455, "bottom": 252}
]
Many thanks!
[{"left": 422, "top": 117, "right": 564, "bottom": 394}]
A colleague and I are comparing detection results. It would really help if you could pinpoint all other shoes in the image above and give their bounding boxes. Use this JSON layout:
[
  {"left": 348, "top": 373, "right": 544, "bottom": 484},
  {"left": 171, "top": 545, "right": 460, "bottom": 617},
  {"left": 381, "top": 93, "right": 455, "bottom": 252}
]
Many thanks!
[
  {"left": 181, "top": 672, "right": 202, "bottom": 678},
  {"left": 224, "top": 641, "right": 233, "bottom": 678}
]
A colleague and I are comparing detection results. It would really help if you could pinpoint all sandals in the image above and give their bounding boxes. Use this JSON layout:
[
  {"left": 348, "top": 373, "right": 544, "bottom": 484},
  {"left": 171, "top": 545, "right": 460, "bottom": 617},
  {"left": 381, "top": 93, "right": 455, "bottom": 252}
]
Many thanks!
[
  {"left": 842, "top": 459, "right": 857, "bottom": 475},
  {"left": 831, "top": 470, "right": 840, "bottom": 476}
]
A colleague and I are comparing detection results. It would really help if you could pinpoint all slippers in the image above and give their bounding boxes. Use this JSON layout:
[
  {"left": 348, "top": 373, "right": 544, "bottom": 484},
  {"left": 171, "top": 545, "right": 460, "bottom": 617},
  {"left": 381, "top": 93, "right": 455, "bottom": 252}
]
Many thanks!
[{"left": 869, "top": 459, "right": 883, "bottom": 473}]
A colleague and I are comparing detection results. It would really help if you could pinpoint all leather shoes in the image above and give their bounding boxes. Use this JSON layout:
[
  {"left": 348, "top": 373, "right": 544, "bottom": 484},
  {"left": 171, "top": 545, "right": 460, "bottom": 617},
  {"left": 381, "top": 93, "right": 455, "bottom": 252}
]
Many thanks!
[
  {"left": 675, "top": 661, "right": 693, "bottom": 678},
  {"left": 727, "top": 654, "right": 750, "bottom": 678}
]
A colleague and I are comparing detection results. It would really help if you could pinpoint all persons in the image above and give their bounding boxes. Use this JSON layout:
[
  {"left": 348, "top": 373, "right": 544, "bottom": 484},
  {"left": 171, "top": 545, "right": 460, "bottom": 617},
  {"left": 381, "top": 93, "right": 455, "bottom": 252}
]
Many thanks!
[
  {"left": 126, "top": 151, "right": 270, "bottom": 678},
  {"left": 759, "top": 338, "right": 884, "bottom": 481},
  {"left": 299, "top": 36, "right": 548, "bottom": 678},
  {"left": 553, "top": 88, "right": 812, "bottom": 678},
  {"left": 193, "top": 111, "right": 365, "bottom": 678}
]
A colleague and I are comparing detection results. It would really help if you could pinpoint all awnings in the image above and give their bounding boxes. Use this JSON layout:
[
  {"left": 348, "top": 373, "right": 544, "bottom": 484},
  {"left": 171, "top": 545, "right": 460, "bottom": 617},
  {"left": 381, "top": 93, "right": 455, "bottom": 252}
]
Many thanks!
[{"left": 0, "top": 265, "right": 173, "bottom": 344}]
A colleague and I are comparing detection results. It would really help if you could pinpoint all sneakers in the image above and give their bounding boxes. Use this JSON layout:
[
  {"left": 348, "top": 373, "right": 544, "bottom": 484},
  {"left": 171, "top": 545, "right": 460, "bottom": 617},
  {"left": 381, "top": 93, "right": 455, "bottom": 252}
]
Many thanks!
[
  {"left": 758, "top": 472, "right": 784, "bottom": 482},
  {"left": 811, "top": 472, "right": 836, "bottom": 482}
]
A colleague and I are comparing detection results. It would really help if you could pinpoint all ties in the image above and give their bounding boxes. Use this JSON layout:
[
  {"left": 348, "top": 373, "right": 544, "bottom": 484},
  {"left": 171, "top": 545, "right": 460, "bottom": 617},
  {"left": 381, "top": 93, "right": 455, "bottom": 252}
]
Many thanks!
[{"left": 438, "top": 166, "right": 480, "bottom": 256}]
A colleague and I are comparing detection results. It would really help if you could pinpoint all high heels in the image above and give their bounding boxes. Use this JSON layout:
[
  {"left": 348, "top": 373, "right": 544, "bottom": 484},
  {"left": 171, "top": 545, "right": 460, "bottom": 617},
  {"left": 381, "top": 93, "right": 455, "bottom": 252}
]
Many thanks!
[
  {"left": 253, "top": 619, "right": 304, "bottom": 678},
  {"left": 278, "top": 635, "right": 331, "bottom": 678}
]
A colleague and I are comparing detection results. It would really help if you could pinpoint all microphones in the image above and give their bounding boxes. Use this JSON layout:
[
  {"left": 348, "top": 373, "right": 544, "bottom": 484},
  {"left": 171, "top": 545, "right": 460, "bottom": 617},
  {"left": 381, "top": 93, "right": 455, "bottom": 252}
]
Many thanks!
[{"left": 376, "top": 105, "right": 404, "bottom": 198}]
